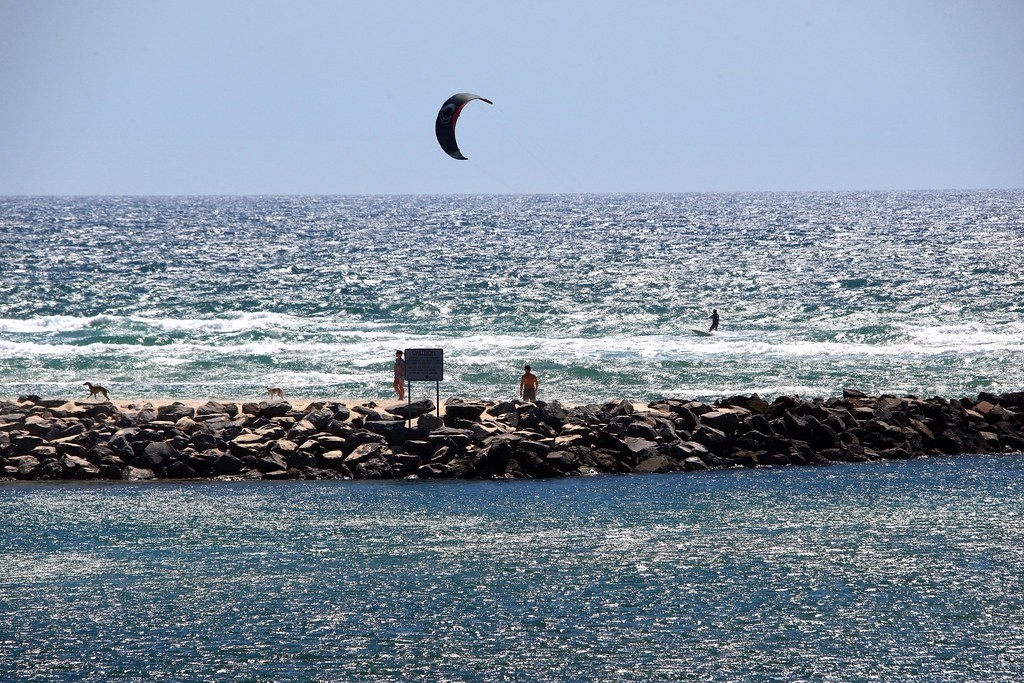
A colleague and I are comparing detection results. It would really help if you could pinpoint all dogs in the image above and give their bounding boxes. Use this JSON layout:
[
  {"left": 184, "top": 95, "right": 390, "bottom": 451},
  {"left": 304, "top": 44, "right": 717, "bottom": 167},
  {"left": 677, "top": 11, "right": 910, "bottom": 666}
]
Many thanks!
[
  {"left": 266, "top": 386, "right": 283, "bottom": 399},
  {"left": 83, "top": 382, "right": 110, "bottom": 401}
]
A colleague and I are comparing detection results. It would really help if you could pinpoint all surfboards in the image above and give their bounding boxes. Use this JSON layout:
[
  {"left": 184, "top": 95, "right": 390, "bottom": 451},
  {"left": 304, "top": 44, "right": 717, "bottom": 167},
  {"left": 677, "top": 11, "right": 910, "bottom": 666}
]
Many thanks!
[{"left": 693, "top": 329, "right": 712, "bottom": 336}]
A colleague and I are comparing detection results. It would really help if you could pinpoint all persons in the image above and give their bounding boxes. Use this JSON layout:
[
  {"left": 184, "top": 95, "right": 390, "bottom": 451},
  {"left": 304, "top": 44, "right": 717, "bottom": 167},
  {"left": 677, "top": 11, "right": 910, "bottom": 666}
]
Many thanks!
[
  {"left": 520, "top": 365, "right": 538, "bottom": 402},
  {"left": 393, "top": 350, "right": 405, "bottom": 401},
  {"left": 706, "top": 309, "right": 719, "bottom": 332}
]
